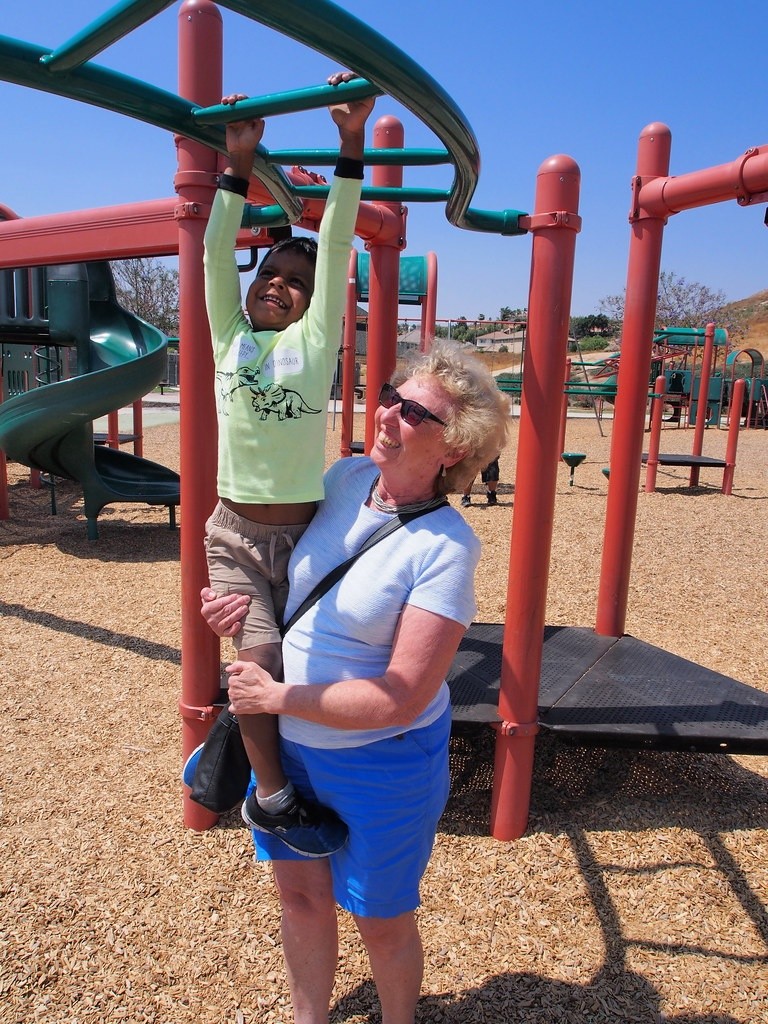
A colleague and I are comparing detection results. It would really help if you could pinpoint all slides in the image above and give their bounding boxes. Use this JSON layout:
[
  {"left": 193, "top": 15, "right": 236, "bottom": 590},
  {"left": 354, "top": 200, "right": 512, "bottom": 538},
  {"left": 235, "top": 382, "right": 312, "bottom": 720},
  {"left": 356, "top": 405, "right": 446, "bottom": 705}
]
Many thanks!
[{"left": 2, "top": 257, "right": 183, "bottom": 542}]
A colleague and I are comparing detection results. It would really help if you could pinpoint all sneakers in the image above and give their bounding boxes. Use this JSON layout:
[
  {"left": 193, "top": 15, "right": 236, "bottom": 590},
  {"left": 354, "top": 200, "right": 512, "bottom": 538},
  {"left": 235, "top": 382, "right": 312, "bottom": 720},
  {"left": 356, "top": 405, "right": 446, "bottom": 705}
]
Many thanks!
[
  {"left": 182, "top": 741, "right": 205, "bottom": 789},
  {"left": 240, "top": 785, "right": 350, "bottom": 858}
]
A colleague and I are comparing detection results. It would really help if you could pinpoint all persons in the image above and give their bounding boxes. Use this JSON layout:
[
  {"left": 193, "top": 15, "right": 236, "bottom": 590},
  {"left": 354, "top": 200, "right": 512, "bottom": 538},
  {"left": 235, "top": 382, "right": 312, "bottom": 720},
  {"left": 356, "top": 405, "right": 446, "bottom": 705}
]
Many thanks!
[
  {"left": 197, "top": 334, "right": 509, "bottom": 1024},
  {"left": 460, "top": 451, "right": 501, "bottom": 508},
  {"left": 202, "top": 68, "right": 379, "bottom": 856}
]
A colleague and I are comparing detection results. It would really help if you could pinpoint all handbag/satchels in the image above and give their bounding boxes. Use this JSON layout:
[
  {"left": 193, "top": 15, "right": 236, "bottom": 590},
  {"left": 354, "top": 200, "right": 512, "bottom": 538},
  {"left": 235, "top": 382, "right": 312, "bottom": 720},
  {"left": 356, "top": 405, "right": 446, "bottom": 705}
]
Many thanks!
[{"left": 190, "top": 701, "right": 251, "bottom": 812}]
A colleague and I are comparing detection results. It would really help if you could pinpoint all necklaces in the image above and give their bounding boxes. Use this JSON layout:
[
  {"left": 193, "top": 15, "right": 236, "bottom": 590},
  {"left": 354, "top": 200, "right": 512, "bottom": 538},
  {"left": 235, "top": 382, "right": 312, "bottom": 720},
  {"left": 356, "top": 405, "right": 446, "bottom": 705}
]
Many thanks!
[{"left": 369, "top": 480, "right": 450, "bottom": 514}]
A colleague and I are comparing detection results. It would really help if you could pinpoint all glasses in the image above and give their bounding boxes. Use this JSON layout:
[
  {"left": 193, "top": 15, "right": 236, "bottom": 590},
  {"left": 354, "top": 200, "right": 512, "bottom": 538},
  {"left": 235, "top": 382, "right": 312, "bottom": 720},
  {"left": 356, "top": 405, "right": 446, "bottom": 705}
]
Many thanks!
[{"left": 377, "top": 383, "right": 449, "bottom": 426}]
